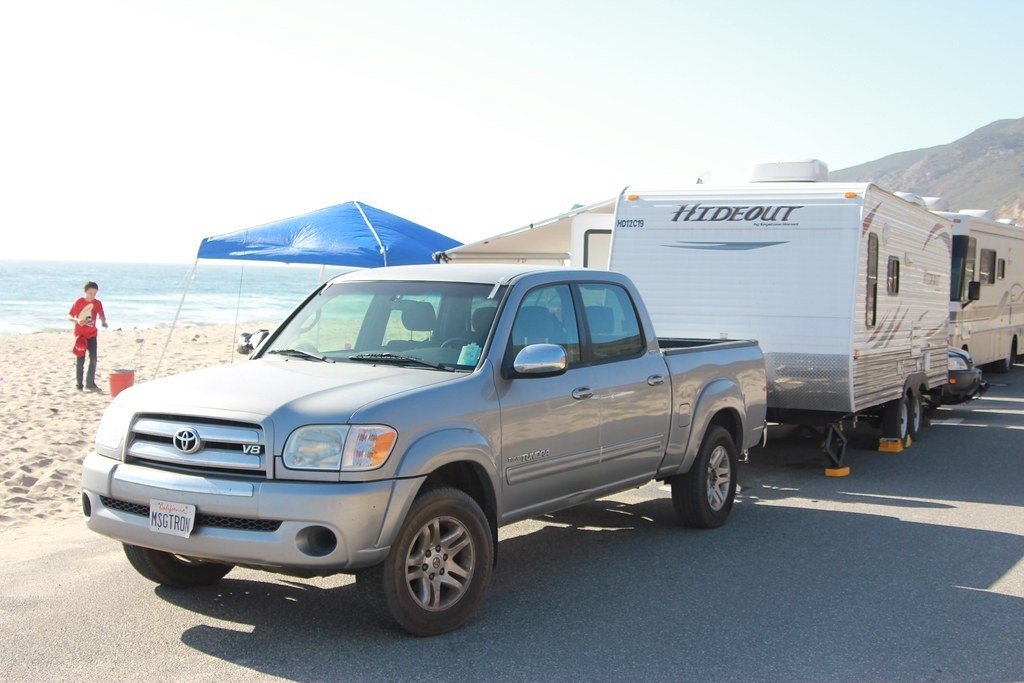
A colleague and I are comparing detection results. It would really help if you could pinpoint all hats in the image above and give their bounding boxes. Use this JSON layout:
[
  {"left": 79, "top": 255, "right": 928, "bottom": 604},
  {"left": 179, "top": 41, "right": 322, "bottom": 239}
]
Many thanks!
[{"left": 72, "top": 335, "right": 88, "bottom": 357}]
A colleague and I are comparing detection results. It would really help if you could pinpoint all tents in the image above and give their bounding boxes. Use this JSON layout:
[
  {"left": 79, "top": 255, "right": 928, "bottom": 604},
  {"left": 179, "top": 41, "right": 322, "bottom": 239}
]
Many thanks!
[{"left": 150, "top": 200, "right": 467, "bottom": 383}]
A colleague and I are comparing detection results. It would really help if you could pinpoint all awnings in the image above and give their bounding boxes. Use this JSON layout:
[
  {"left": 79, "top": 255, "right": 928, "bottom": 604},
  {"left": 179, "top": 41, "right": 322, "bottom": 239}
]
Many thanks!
[{"left": 432, "top": 194, "right": 618, "bottom": 266}]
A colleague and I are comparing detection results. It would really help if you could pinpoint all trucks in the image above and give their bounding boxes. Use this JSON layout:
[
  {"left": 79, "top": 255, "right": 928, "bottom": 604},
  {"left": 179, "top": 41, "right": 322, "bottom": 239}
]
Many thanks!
[{"left": 567, "top": 158, "right": 1024, "bottom": 451}]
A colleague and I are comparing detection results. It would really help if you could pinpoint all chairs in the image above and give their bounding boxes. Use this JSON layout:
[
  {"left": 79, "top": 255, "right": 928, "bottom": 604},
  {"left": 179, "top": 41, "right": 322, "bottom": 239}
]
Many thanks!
[
  {"left": 569, "top": 306, "right": 618, "bottom": 352},
  {"left": 469, "top": 307, "right": 498, "bottom": 348},
  {"left": 387, "top": 302, "right": 442, "bottom": 351},
  {"left": 513, "top": 306, "right": 555, "bottom": 366}
]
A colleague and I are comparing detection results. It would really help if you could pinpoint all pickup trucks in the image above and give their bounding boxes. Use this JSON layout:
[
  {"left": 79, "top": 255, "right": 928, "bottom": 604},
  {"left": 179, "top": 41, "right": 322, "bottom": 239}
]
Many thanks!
[{"left": 77, "top": 261, "right": 769, "bottom": 636}]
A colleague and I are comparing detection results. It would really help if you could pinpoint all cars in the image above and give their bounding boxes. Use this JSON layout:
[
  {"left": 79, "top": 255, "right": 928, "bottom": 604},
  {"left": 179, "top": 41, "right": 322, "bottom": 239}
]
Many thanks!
[{"left": 924, "top": 346, "right": 989, "bottom": 416}]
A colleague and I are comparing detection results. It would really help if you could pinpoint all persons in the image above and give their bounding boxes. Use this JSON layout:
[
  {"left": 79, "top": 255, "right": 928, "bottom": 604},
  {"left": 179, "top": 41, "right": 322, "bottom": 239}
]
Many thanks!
[{"left": 69, "top": 281, "right": 108, "bottom": 391}]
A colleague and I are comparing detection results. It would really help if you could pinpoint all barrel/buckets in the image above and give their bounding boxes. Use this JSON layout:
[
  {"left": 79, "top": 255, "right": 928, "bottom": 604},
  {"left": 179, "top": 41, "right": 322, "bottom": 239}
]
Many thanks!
[{"left": 108, "top": 372, "right": 135, "bottom": 398}]
